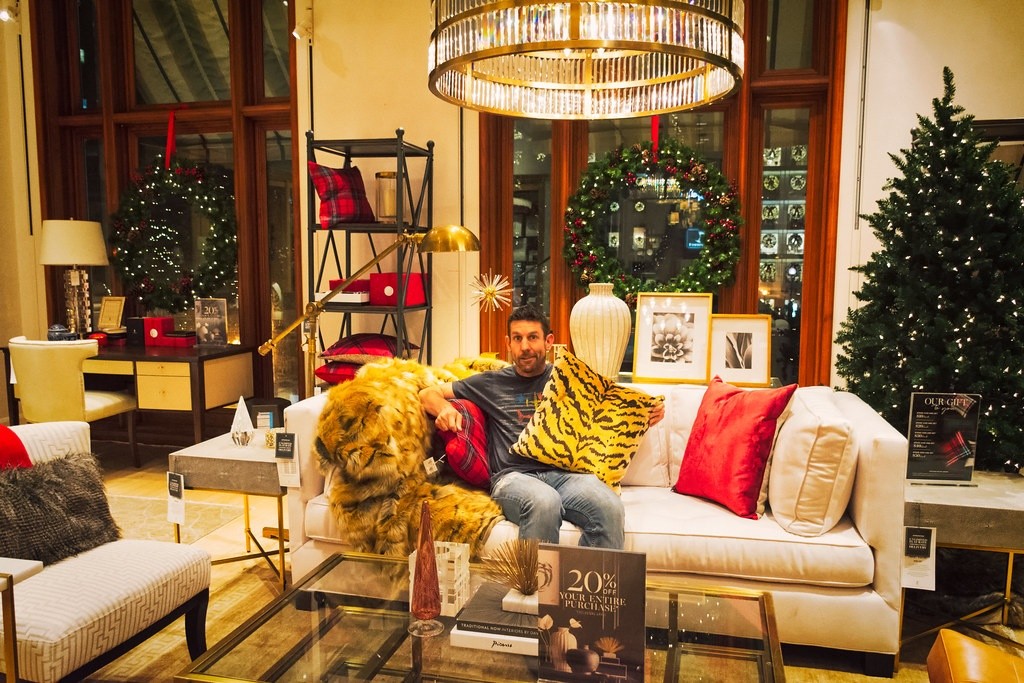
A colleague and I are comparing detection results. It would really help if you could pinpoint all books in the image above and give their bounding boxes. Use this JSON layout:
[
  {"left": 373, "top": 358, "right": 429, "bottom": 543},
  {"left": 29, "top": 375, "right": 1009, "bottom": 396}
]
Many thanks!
[{"left": 449, "top": 581, "right": 538, "bottom": 656}]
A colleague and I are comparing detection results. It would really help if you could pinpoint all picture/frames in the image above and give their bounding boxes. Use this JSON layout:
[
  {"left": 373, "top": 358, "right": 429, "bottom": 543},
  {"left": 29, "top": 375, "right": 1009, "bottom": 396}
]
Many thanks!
[
  {"left": 98, "top": 297, "right": 125, "bottom": 327},
  {"left": 708, "top": 313, "right": 772, "bottom": 388},
  {"left": 632, "top": 292, "right": 712, "bottom": 384}
]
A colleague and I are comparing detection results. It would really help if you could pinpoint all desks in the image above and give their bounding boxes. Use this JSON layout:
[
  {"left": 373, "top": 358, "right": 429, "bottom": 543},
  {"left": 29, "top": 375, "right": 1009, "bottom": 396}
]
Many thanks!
[
  {"left": 0, "top": 556, "right": 43, "bottom": 683},
  {"left": 0, "top": 343, "right": 261, "bottom": 444},
  {"left": 168, "top": 428, "right": 289, "bottom": 591},
  {"left": 895, "top": 471, "right": 1024, "bottom": 672},
  {"left": 618, "top": 372, "right": 782, "bottom": 388}
]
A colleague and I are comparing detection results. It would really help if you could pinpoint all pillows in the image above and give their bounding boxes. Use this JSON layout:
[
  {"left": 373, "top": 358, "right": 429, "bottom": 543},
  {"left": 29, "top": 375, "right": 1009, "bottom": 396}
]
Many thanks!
[
  {"left": 670, "top": 375, "right": 800, "bottom": 518},
  {"left": 437, "top": 398, "right": 491, "bottom": 487},
  {"left": 314, "top": 360, "right": 362, "bottom": 386},
  {"left": 318, "top": 333, "right": 421, "bottom": 365},
  {"left": 307, "top": 161, "right": 380, "bottom": 231},
  {"left": 0, "top": 450, "right": 123, "bottom": 568},
  {"left": 508, "top": 346, "right": 663, "bottom": 496}
]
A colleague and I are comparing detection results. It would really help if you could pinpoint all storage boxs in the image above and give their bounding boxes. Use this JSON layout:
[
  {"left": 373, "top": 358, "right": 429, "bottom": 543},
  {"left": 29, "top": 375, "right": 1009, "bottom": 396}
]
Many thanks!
[
  {"left": 369, "top": 272, "right": 426, "bottom": 308},
  {"left": 314, "top": 292, "right": 369, "bottom": 307}
]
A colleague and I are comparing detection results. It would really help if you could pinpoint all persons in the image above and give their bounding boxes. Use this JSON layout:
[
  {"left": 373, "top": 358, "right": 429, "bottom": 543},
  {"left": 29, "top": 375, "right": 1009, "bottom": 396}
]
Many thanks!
[{"left": 418, "top": 304, "right": 665, "bottom": 548}]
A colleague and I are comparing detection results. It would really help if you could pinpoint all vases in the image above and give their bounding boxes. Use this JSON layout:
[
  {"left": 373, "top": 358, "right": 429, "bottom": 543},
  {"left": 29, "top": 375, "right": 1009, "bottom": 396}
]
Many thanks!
[{"left": 569, "top": 283, "right": 631, "bottom": 381}]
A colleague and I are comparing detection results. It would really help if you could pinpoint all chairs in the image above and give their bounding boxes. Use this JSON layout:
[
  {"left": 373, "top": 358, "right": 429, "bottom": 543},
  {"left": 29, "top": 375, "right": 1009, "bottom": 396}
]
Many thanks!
[
  {"left": 0, "top": 421, "right": 211, "bottom": 683},
  {"left": 8, "top": 336, "right": 142, "bottom": 469}
]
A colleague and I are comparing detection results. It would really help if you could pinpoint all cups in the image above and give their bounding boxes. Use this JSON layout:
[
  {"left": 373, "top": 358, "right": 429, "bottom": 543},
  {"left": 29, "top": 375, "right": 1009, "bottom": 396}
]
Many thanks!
[
  {"left": 47, "top": 323, "right": 67, "bottom": 340},
  {"left": 62, "top": 332, "right": 80, "bottom": 341}
]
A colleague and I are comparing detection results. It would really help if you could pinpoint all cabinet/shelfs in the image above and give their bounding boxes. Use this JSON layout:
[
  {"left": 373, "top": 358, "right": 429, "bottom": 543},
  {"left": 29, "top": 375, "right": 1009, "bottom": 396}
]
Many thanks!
[{"left": 305, "top": 127, "right": 433, "bottom": 393}]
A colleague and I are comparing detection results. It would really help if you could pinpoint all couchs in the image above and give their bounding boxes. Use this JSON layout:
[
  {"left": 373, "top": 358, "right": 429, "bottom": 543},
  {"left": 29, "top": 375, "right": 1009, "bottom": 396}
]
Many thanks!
[
  {"left": 284, "top": 356, "right": 910, "bottom": 678},
  {"left": 927, "top": 629, "right": 1024, "bottom": 683}
]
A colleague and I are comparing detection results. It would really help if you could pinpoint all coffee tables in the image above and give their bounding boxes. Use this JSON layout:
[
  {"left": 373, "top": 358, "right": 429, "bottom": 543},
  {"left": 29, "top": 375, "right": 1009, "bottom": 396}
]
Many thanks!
[{"left": 172, "top": 552, "right": 790, "bottom": 683}]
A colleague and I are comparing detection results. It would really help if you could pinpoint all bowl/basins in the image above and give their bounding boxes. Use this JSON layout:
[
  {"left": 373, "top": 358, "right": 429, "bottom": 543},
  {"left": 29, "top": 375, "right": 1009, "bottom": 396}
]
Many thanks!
[{"left": 566, "top": 649, "right": 599, "bottom": 674}]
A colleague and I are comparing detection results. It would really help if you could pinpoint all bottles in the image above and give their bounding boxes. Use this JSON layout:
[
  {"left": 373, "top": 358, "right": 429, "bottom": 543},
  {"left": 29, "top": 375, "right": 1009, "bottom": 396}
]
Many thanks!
[{"left": 551, "top": 627, "right": 577, "bottom": 672}]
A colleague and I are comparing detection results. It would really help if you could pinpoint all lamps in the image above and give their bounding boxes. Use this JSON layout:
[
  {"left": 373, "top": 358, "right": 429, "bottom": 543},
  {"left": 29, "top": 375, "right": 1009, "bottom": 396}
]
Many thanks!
[
  {"left": 427, "top": 0, "right": 747, "bottom": 121},
  {"left": 258, "top": 224, "right": 483, "bottom": 399},
  {"left": 39, "top": 217, "right": 110, "bottom": 340}
]
